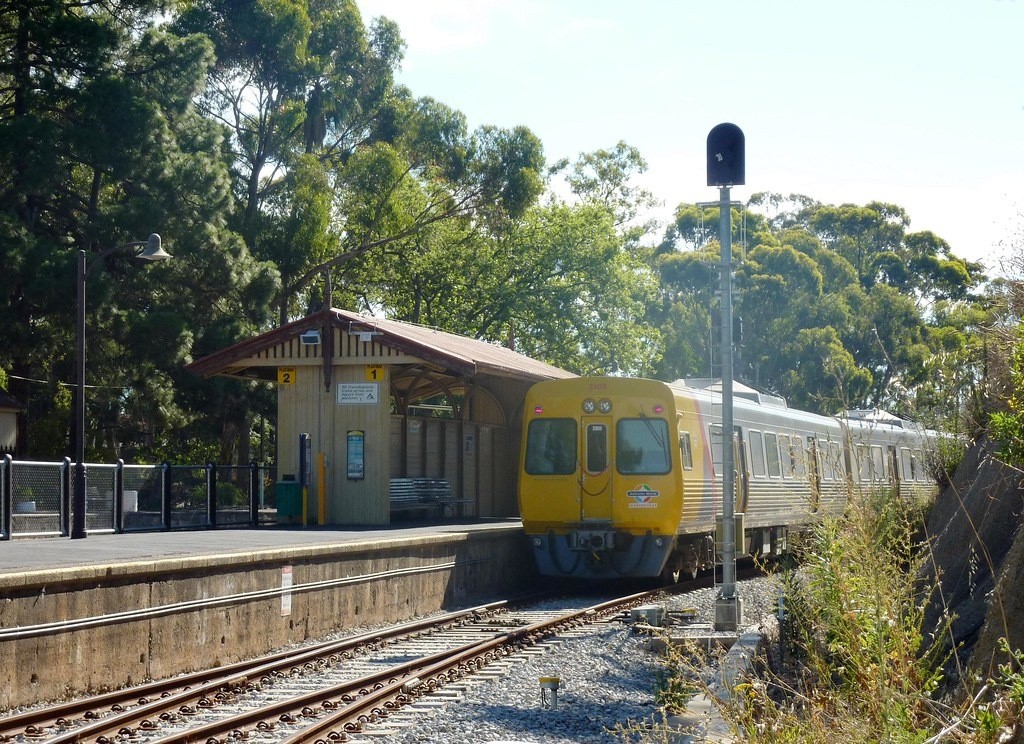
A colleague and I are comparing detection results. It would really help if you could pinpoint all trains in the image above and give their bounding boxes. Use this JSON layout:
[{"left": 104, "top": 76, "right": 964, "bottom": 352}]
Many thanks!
[{"left": 518, "top": 375, "right": 972, "bottom": 592}]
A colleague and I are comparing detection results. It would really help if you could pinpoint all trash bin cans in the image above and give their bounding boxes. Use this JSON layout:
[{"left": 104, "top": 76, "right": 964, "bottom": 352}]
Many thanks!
[{"left": 274, "top": 473, "right": 303, "bottom": 524}]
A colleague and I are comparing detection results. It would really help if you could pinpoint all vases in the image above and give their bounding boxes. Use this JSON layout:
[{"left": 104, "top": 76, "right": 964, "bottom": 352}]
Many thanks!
[{"left": 15, "top": 484, "right": 36, "bottom": 514}]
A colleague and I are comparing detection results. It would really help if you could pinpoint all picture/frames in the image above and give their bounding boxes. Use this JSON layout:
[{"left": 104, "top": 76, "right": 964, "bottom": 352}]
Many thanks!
[{"left": 347, "top": 430, "right": 365, "bottom": 480}]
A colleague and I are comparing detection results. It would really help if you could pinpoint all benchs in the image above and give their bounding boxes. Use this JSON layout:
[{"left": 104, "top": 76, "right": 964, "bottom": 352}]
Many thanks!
[
  {"left": 86, "top": 487, "right": 112, "bottom": 513},
  {"left": 390, "top": 478, "right": 476, "bottom": 528}
]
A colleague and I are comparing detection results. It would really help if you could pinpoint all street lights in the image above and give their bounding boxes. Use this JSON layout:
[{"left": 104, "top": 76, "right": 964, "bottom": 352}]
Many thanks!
[{"left": 69, "top": 232, "right": 173, "bottom": 538}]
